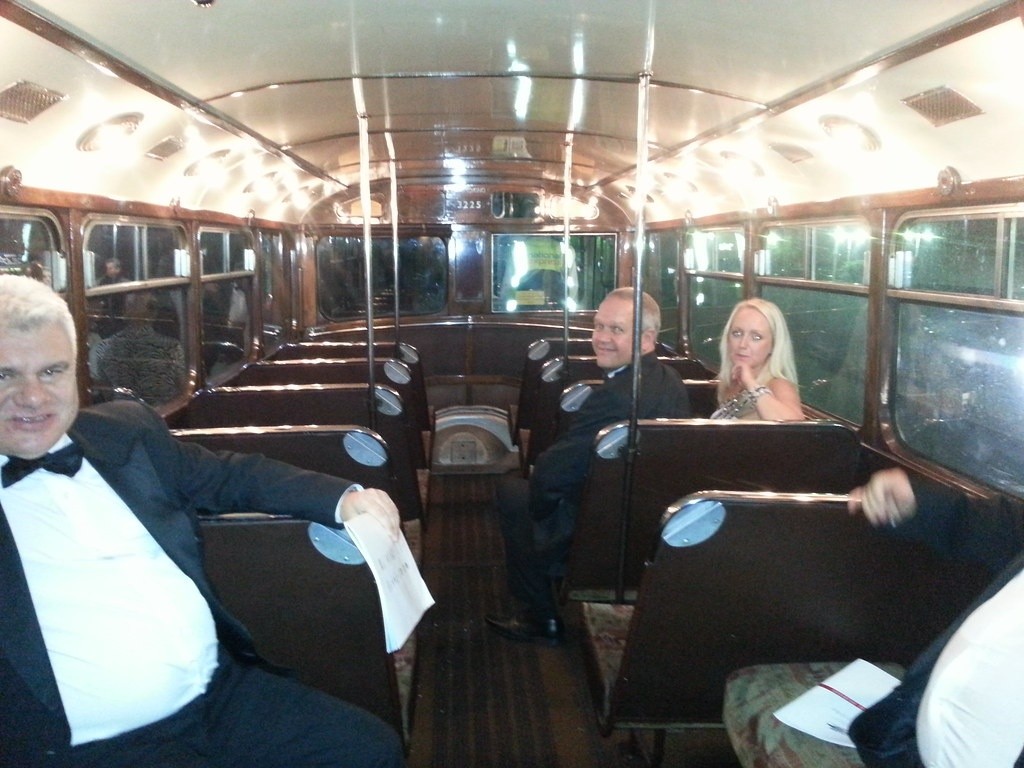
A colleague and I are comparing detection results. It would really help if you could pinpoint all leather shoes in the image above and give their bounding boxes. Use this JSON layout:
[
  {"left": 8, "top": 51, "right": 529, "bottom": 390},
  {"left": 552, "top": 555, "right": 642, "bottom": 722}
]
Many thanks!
[{"left": 484, "top": 608, "right": 562, "bottom": 647}]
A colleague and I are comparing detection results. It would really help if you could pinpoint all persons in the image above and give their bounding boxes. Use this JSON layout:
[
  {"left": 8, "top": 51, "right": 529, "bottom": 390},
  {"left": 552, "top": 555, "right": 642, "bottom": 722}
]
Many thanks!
[
  {"left": 487, "top": 289, "right": 690, "bottom": 642},
  {"left": 708, "top": 296, "right": 808, "bottom": 420},
  {"left": 849, "top": 464, "right": 1024, "bottom": 768},
  {"left": 87, "top": 257, "right": 247, "bottom": 406},
  {"left": 1, "top": 273, "right": 404, "bottom": 768}
]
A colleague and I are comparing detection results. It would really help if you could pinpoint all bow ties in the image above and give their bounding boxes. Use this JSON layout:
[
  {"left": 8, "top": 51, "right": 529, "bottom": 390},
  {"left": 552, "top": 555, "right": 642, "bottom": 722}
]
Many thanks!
[{"left": 0, "top": 440, "right": 84, "bottom": 488}]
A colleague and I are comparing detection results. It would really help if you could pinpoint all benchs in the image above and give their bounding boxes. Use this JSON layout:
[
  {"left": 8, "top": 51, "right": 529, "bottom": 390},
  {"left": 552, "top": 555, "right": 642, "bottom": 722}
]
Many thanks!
[
  {"left": 166, "top": 339, "right": 437, "bottom": 759},
  {"left": 504, "top": 337, "right": 970, "bottom": 768}
]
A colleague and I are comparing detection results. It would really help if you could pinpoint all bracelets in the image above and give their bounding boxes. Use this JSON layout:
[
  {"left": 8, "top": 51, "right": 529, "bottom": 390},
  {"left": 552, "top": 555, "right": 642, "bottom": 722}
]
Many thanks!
[{"left": 750, "top": 386, "right": 775, "bottom": 398}]
